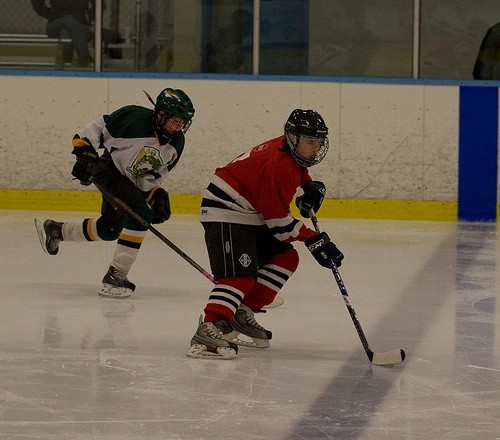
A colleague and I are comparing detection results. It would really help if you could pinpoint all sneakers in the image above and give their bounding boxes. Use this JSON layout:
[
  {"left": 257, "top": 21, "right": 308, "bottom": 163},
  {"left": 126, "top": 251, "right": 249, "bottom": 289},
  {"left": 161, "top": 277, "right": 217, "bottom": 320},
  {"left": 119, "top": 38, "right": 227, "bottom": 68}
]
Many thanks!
[
  {"left": 186, "top": 314, "right": 239, "bottom": 360},
  {"left": 97, "top": 265, "right": 136, "bottom": 299},
  {"left": 35, "top": 217, "right": 67, "bottom": 256},
  {"left": 222, "top": 304, "right": 272, "bottom": 348}
]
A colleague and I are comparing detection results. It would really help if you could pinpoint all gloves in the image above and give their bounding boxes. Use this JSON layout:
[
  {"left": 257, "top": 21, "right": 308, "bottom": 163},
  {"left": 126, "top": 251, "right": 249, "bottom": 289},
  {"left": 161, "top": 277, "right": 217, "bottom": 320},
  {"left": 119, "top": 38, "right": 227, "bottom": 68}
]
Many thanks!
[
  {"left": 71, "top": 152, "right": 98, "bottom": 186},
  {"left": 296, "top": 182, "right": 327, "bottom": 219},
  {"left": 148, "top": 189, "right": 171, "bottom": 225},
  {"left": 304, "top": 232, "right": 344, "bottom": 269}
]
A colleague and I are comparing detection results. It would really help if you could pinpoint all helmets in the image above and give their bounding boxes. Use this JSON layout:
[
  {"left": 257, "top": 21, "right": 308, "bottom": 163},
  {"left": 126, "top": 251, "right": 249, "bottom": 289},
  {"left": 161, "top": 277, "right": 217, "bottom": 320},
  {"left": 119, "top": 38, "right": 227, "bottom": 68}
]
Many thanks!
[
  {"left": 154, "top": 88, "right": 195, "bottom": 139},
  {"left": 284, "top": 109, "right": 330, "bottom": 169}
]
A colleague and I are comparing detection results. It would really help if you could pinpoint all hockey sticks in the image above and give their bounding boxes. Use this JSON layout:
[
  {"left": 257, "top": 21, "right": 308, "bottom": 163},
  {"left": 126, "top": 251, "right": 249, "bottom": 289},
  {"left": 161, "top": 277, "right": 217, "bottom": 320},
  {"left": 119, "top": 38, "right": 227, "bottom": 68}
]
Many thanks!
[
  {"left": 88, "top": 175, "right": 284, "bottom": 309},
  {"left": 308, "top": 209, "right": 406, "bottom": 365}
]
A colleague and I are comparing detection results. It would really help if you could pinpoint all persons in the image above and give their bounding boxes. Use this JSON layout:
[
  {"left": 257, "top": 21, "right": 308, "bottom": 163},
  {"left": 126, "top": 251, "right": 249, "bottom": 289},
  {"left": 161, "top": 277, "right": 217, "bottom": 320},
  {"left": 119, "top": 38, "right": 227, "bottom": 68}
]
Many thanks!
[
  {"left": 473, "top": 21, "right": 500, "bottom": 80},
  {"left": 207, "top": 9, "right": 270, "bottom": 76},
  {"left": 31, "top": 0, "right": 96, "bottom": 67},
  {"left": 190, "top": 108, "right": 344, "bottom": 354},
  {"left": 124, "top": 11, "right": 170, "bottom": 74},
  {"left": 43, "top": 87, "right": 195, "bottom": 291}
]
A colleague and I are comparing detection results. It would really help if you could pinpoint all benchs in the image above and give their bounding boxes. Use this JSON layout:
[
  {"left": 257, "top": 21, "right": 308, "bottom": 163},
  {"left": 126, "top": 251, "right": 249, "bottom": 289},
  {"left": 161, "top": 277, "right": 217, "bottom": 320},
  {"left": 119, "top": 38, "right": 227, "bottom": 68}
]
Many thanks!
[{"left": 0, "top": 33, "right": 135, "bottom": 67}]
